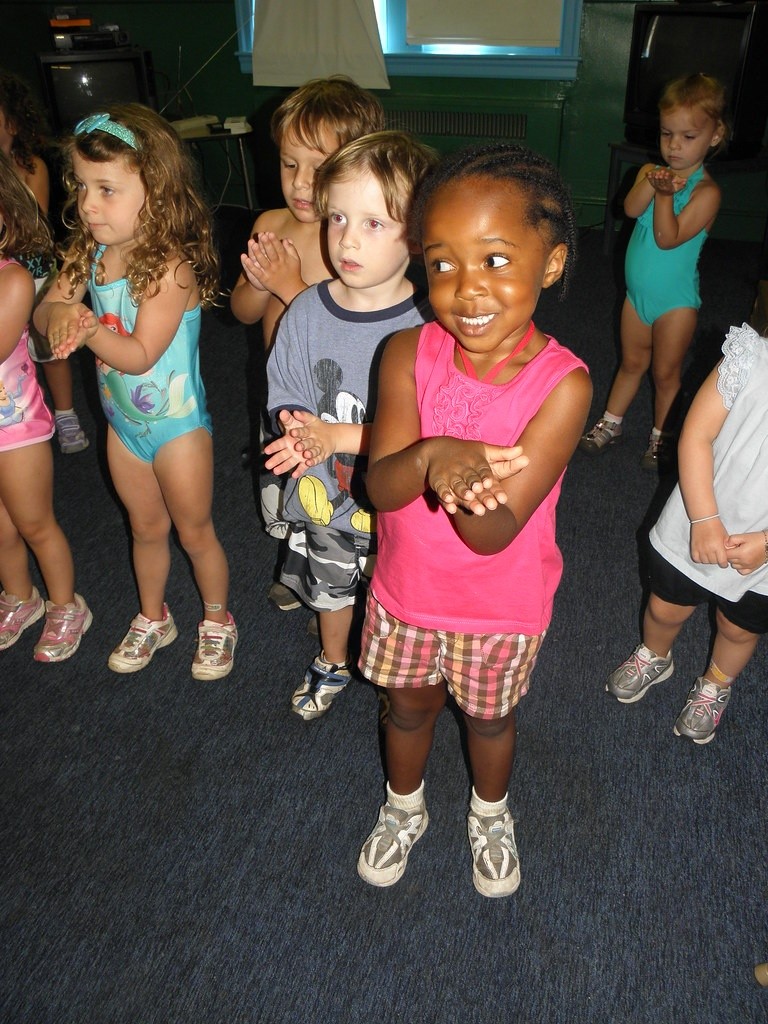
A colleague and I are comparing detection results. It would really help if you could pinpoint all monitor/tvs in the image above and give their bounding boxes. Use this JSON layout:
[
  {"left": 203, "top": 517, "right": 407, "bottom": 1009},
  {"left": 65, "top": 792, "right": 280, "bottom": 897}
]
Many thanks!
[{"left": 38, "top": 49, "right": 155, "bottom": 134}]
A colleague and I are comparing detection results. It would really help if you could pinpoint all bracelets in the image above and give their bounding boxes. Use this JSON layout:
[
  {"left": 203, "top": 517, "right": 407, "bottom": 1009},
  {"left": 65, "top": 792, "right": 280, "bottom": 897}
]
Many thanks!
[
  {"left": 761, "top": 529, "right": 768, "bottom": 564},
  {"left": 691, "top": 514, "right": 719, "bottom": 524}
]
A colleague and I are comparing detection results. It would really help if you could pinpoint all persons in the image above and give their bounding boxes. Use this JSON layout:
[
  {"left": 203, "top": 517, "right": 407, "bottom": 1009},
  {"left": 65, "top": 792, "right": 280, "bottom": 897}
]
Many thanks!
[
  {"left": 0, "top": 66, "right": 90, "bottom": 458},
  {"left": 355, "top": 147, "right": 596, "bottom": 898},
  {"left": 0, "top": 153, "right": 95, "bottom": 665},
  {"left": 230, "top": 72, "right": 386, "bottom": 638},
  {"left": 33, "top": 102, "right": 239, "bottom": 681},
  {"left": 598, "top": 276, "right": 768, "bottom": 747},
  {"left": 264, "top": 127, "right": 444, "bottom": 723},
  {"left": 577, "top": 72, "right": 733, "bottom": 473}
]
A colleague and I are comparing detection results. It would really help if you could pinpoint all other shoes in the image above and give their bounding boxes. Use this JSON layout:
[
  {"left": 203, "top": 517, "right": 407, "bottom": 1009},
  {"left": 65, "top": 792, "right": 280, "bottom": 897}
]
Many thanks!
[{"left": 259, "top": 488, "right": 295, "bottom": 541}]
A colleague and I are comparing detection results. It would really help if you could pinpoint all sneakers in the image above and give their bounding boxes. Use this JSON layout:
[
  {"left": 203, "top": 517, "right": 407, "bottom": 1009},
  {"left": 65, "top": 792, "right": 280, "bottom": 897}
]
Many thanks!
[
  {"left": 32, "top": 592, "right": 93, "bottom": 663},
  {"left": 607, "top": 643, "right": 675, "bottom": 704},
  {"left": 289, "top": 647, "right": 355, "bottom": 723},
  {"left": 0, "top": 586, "right": 46, "bottom": 652},
  {"left": 640, "top": 435, "right": 675, "bottom": 481},
  {"left": 356, "top": 804, "right": 429, "bottom": 888},
  {"left": 305, "top": 609, "right": 323, "bottom": 641},
  {"left": 268, "top": 579, "right": 305, "bottom": 612},
  {"left": 189, "top": 610, "right": 241, "bottom": 681},
  {"left": 466, "top": 807, "right": 521, "bottom": 899},
  {"left": 672, "top": 675, "right": 732, "bottom": 745},
  {"left": 53, "top": 411, "right": 90, "bottom": 455},
  {"left": 577, "top": 418, "right": 624, "bottom": 457},
  {"left": 106, "top": 602, "right": 181, "bottom": 675}
]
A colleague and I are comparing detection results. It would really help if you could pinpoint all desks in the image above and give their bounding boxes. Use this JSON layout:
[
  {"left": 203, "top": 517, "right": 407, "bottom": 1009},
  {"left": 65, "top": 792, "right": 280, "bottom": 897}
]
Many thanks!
[
  {"left": 604, "top": 141, "right": 768, "bottom": 251},
  {"left": 32, "top": 120, "right": 257, "bottom": 224}
]
